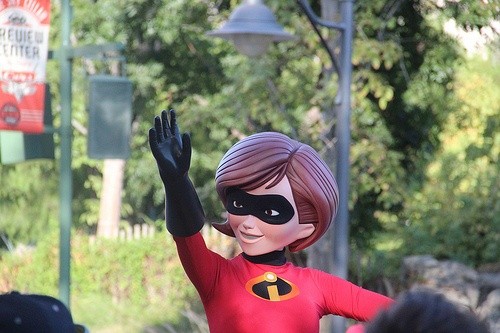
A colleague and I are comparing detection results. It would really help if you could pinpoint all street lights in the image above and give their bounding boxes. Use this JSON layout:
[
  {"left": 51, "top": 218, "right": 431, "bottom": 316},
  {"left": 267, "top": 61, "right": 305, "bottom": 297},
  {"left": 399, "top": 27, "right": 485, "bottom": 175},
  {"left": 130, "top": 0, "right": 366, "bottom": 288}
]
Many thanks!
[{"left": 206, "top": 0, "right": 354, "bottom": 333}]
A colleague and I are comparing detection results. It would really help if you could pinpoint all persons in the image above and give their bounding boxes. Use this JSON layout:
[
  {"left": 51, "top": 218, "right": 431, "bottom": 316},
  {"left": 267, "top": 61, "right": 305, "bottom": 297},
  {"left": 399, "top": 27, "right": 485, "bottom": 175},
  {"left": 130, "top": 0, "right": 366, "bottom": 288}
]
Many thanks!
[
  {"left": 0, "top": 289, "right": 90, "bottom": 333},
  {"left": 343, "top": 285, "right": 491, "bottom": 333},
  {"left": 148, "top": 108, "right": 400, "bottom": 333}
]
